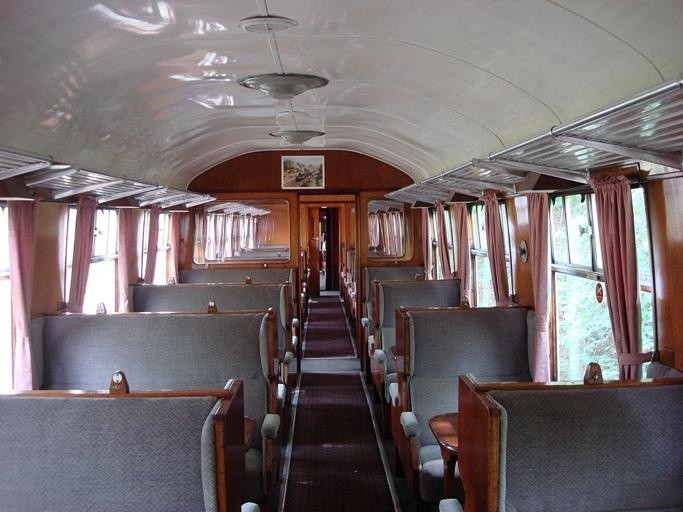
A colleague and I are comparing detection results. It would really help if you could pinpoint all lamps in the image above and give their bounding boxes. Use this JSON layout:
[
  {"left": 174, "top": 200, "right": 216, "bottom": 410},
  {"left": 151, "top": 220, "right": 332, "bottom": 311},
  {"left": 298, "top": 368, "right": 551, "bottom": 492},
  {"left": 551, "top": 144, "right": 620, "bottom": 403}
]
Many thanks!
[
  {"left": 269, "top": 131, "right": 326, "bottom": 144},
  {"left": 238, "top": 73, "right": 329, "bottom": 100}
]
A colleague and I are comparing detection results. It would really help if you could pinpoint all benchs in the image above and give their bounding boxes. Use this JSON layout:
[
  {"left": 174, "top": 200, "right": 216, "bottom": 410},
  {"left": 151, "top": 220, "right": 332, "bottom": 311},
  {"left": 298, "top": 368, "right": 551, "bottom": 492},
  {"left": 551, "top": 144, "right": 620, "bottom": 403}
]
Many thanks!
[
  {"left": 340, "top": 247, "right": 683, "bottom": 512},
  {"left": 0, "top": 245, "right": 309, "bottom": 512}
]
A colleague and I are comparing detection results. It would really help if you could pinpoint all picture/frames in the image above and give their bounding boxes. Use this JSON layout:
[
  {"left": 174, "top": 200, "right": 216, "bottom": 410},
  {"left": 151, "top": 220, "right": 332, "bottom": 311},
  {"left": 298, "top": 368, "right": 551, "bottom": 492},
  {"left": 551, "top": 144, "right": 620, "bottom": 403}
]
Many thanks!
[{"left": 281, "top": 155, "right": 325, "bottom": 190}]
